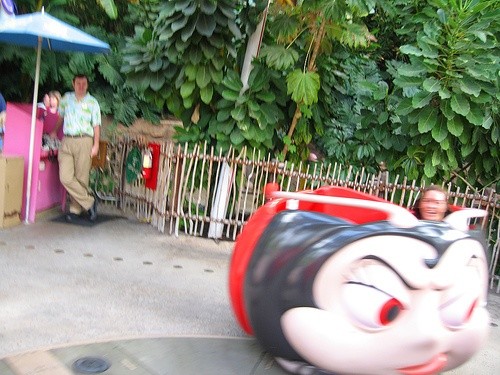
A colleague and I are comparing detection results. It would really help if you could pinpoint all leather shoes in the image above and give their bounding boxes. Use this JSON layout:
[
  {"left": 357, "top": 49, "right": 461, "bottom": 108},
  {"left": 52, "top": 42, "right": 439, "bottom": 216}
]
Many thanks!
[
  {"left": 67, "top": 212, "right": 82, "bottom": 223},
  {"left": 87, "top": 196, "right": 97, "bottom": 221}
]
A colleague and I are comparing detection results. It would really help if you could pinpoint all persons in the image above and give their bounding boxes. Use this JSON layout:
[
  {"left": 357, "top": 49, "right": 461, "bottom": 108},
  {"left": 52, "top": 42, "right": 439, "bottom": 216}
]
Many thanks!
[
  {"left": 414, "top": 186, "right": 453, "bottom": 222},
  {"left": 49, "top": 74, "right": 102, "bottom": 222},
  {"left": 35, "top": 90, "right": 63, "bottom": 154}
]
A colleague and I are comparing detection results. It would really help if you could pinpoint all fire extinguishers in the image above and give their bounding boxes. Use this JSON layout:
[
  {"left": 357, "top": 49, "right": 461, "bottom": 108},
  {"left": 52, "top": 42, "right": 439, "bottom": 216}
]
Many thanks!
[{"left": 140, "top": 145, "right": 152, "bottom": 181}]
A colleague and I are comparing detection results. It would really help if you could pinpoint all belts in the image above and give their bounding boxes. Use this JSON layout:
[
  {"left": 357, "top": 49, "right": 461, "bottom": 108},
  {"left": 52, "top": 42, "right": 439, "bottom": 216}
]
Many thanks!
[{"left": 64, "top": 134, "right": 85, "bottom": 138}]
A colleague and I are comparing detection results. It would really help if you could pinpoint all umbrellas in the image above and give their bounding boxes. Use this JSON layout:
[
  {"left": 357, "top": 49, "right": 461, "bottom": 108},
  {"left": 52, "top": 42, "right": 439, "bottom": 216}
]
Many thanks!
[{"left": 0, "top": 4, "right": 110, "bottom": 224}]
